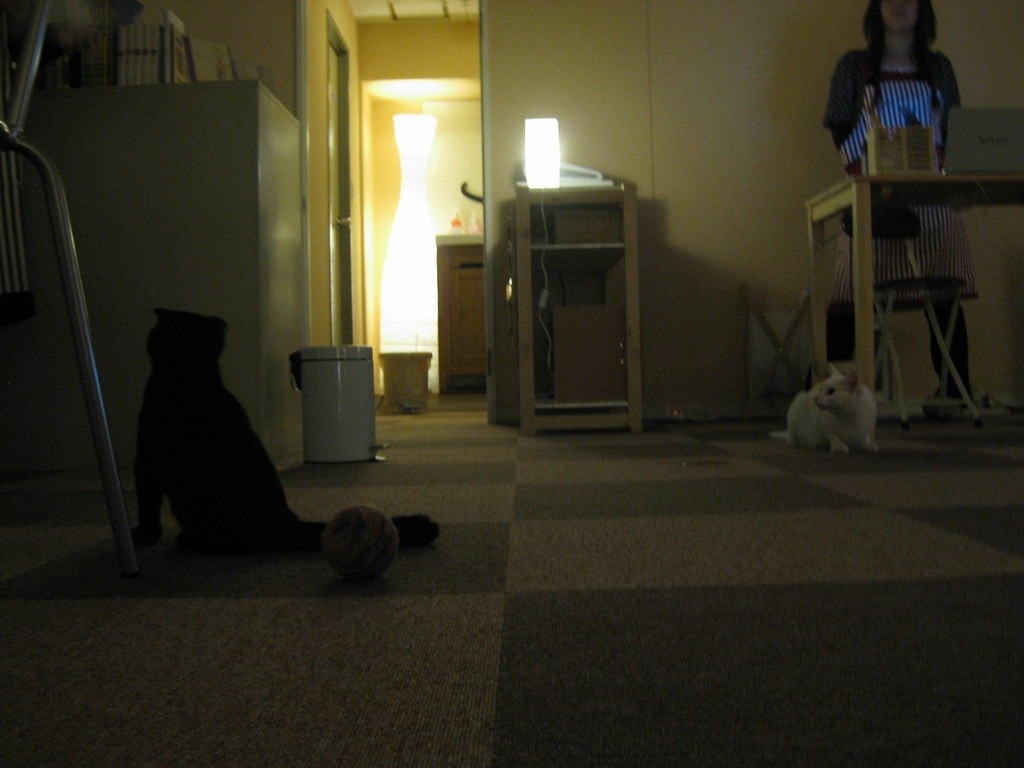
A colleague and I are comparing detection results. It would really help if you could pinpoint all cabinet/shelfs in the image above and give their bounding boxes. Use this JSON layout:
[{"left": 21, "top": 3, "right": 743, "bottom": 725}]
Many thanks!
[{"left": 515, "top": 178, "right": 642, "bottom": 435}]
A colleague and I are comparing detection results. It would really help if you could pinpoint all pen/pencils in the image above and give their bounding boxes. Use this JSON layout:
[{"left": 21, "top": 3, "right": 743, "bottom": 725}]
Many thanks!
[{"left": 867, "top": 104, "right": 888, "bottom": 140}]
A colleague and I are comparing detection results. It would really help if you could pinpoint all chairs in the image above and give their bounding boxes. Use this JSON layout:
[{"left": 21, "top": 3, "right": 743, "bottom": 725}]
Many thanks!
[{"left": 840, "top": 206, "right": 983, "bottom": 430}]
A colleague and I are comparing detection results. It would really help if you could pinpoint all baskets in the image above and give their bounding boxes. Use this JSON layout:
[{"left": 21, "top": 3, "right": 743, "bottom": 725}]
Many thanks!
[
  {"left": 867, "top": 126, "right": 935, "bottom": 176},
  {"left": 553, "top": 210, "right": 621, "bottom": 243}
]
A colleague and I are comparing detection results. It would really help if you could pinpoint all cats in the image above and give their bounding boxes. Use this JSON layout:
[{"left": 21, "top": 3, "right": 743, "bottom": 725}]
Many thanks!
[
  {"left": 127, "top": 305, "right": 441, "bottom": 557},
  {"left": 768, "top": 363, "right": 878, "bottom": 455}
]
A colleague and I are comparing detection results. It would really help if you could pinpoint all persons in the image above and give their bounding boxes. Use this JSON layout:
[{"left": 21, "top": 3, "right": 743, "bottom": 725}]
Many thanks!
[{"left": 822, "top": 0, "right": 972, "bottom": 423}]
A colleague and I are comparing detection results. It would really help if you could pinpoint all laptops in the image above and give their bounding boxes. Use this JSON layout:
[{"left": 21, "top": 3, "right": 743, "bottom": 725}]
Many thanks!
[{"left": 942, "top": 108, "right": 1024, "bottom": 175}]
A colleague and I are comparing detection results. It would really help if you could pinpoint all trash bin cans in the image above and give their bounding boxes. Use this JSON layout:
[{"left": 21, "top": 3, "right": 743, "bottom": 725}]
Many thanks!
[
  {"left": 377, "top": 352, "right": 433, "bottom": 415},
  {"left": 299, "top": 345, "right": 391, "bottom": 463}
]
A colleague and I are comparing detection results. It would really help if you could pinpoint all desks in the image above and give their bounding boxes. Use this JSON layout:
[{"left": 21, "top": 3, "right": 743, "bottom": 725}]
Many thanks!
[
  {"left": 435, "top": 234, "right": 484, "bottom": 396},
  {"left": 805, "top": 173, "right": 1024, "bottom": 399}
]
